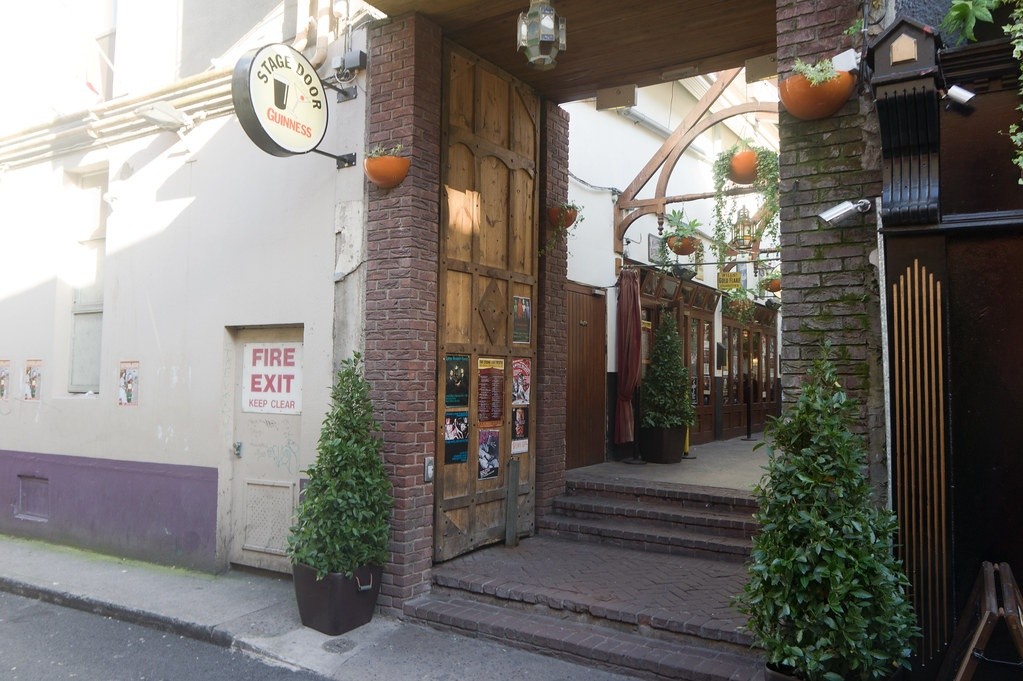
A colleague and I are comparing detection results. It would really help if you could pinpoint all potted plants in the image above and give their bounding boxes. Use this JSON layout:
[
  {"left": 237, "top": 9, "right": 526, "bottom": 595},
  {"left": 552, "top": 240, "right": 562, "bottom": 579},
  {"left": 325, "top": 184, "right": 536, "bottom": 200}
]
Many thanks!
[
  {"left": 287, "top": 352, "right": 393, "bottom": 635},
  {"left": 658, "top": 139, "right": 781, "bottom": 325},
  {"left": 729, "top": 338, "right": 923, "bottom": 681},
  {"left": 534, "top": 202, "right": 584, "bottom": 256},
  {"left": 363, "top": 144, "right": 410, "bottom": 188},
  {"left": 639, "top": 304, "right": 694, "bottom": 464},
  {"left": 779, "top": 58, "right": 855, "bottom": 120}
]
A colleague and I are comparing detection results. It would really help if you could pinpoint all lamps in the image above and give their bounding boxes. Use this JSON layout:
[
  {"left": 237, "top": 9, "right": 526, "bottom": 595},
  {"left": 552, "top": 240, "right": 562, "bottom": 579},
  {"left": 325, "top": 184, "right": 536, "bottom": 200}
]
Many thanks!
[
  {"left": 765, "top": 298, "right": 781, "bottom": 310},
  {"left": 517, "top": 0, "right": 567, "bottom": 66},
  {"left": 672, "top": 263, "right": 697, "bottom": 281}
]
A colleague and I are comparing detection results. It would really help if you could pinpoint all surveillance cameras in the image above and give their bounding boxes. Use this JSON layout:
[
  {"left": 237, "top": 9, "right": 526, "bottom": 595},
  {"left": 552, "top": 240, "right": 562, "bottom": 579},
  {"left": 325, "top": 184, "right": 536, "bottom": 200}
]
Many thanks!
[
  {"left": 818, "top": 201, "right": 857, "bottom": 228},
  {"left": 946, "top": 84, "right": 976, "bottom": 104}
]
